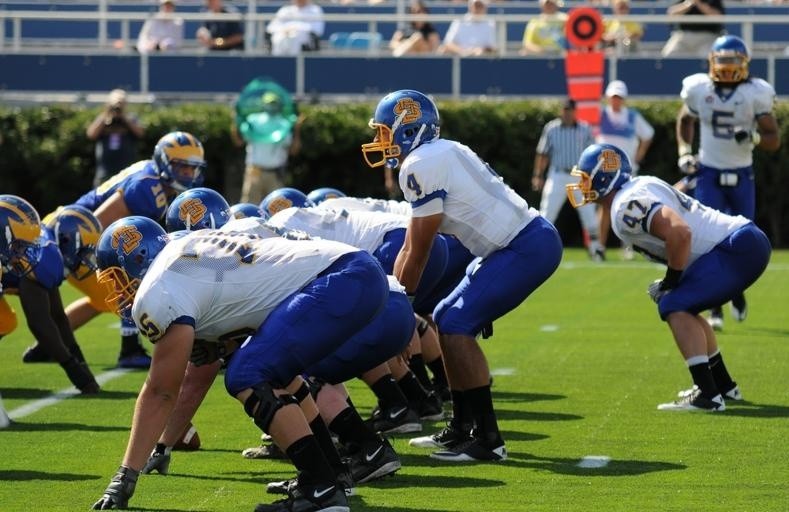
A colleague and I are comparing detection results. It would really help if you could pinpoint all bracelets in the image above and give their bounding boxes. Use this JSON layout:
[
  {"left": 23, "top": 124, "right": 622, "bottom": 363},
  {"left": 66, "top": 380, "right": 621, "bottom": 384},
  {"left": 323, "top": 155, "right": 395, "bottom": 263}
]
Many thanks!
[{"left": 534, "top": 171, "right": 544, "bottom": 179}]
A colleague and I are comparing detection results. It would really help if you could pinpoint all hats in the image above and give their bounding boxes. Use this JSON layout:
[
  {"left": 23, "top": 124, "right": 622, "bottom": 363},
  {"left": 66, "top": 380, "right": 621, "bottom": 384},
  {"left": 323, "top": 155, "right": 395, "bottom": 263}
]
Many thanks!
[
  {"left": 563, "top": 100, "right": 575, "bottom": 111},
  {"left": 261, "top": 92, "right": 280, "bottom": 104},
  {"left": 605, "top": 80, "right": 628, "bottom": 100}
]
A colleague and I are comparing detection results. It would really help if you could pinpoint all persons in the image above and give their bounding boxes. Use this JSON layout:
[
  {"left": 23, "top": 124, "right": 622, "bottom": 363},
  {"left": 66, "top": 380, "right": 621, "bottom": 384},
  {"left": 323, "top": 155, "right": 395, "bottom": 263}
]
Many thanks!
[
  {"left": 1, "top": 194, "right": 102, "bottom": 428},
  {"left": 566, "top": 143, "right": 771, "bottom": 413},
  {"left": 18, "top": 130, "right": 207, "bottom": 369},
  {"left": 674, "top": 37, "right": 782, "bottom": 330},
  {"left": 86, "top": 86, "right": 145, "bottom": 189},
  {"left": 390, "top": 0, "right": 497, "bottom": 54},
  {"left": 522, "top": 1, "right": 643, "bottom": 53},
  {"left": 229, "top": 90, "right": 305, "bottom": 206},
  {"left": 93, "top": 214, "right": 390, "bottom": 512},
  {"left": 166, "top": 185, "right": 414, "bottom": 492},
  {"left": 663, "top": 1, "right": 728, "bottom": 56},
  {"left": 594, "top": 78, "right": 658, "bottom": 260},
  {"left": 137, "top": 0, "right": 327, "bottom": 52},
  {"left": 530, "top": 95, "right": 600, "bottom": 260},
  {"left": 362, "top": 90, "right": 563, "bottom": 462}
]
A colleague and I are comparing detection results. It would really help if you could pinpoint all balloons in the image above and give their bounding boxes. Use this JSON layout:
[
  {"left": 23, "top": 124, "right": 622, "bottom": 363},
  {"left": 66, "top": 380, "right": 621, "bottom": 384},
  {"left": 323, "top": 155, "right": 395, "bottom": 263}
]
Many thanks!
[{"left": 232, "top": 74, "right": 298, "bottom": 142}]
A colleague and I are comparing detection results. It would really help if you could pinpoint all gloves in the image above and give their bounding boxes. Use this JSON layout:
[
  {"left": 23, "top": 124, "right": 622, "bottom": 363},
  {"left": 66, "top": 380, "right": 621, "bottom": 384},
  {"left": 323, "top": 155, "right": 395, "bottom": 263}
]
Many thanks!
[
  {"left": 677, "top": 148, "right": 697, "bottom": 175},
  {"left": 92, "top": 466, "right": 139, "bottom": 510},
  {"left": 141, "top": 444, "right": 172, "bottom": 475},
  {"left": 734, "top": 127, "right": 761, "bottom": 145},
  {"left": 647, "top": 279, "right": 670, "bottom": 304}
]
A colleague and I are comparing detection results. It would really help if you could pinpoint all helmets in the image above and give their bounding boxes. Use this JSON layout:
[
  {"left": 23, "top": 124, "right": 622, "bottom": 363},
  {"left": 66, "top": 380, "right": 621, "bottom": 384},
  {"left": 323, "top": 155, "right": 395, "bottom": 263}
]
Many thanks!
[
  {"left": 565, "top": 144, "right": 632, "bottom": 209},
  {"left": 709, "top": 35, "right": 749, "bottom": 83},
  {"left": 153, "top": 130, "right": 204, "bottom": 185},
  {"left": 1, "top": 185, "right": 345, "bottom": 280},
  {"left": 361, "top": 89, "right": 441, "bottom": 167}
]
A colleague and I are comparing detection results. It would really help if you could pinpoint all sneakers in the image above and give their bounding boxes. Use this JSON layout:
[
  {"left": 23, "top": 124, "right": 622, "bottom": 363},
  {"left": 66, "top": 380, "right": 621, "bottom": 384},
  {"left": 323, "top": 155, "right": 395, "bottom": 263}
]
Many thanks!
[
  {"left": 707, "top": 317, "right": 724, "bottom": 328},
  {"left": 244, "top": 384, "right": 507, "bottom": 512},
  {"left": 23, "top": 346, "right": 56, "bottom": 362},
  {"left": 658, "top": 389, "right": 738, "bottom": 411},
  {"left": 116, "top": 351, "right": 150, "bottom": 367},
  {"left": 734, "top": 310, "right": 746, "bottom": 321}
]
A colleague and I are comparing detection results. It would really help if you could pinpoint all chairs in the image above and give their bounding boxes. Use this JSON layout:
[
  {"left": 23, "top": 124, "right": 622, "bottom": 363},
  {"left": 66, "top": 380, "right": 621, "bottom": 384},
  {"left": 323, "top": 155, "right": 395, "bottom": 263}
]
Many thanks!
[{"left": 345, "top": 31, "right": 381, "bottom": 53}]
